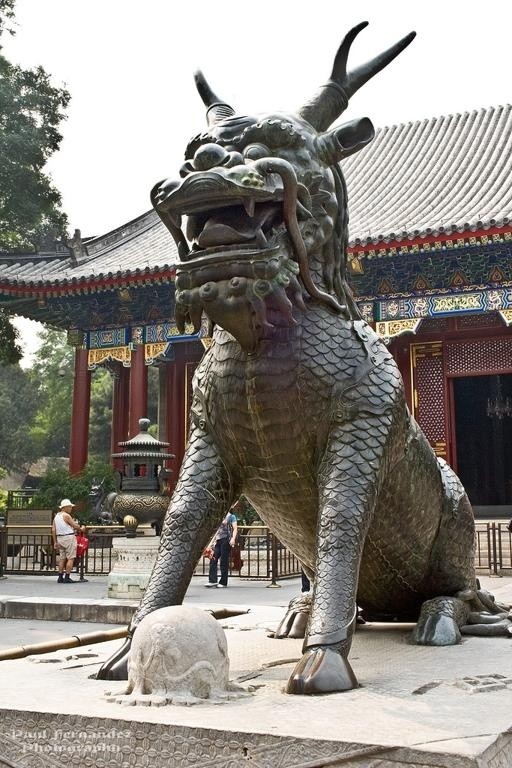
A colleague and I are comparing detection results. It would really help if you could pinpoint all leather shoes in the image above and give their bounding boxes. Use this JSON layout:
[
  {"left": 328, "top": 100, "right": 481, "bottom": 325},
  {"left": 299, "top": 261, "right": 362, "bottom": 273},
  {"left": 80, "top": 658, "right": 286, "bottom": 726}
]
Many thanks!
[
  {"left": 58, "top": 577, "right": 64, "bottom": 582},
  {"left": 63, "top": 578, "right": 74, "bottom": 583}
]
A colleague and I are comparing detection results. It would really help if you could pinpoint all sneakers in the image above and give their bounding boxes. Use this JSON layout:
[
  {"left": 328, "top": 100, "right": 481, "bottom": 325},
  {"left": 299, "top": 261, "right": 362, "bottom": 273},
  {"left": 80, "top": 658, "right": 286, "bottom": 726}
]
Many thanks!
[
  {"left": 204, "top": 582, "right": 218, "bottom": 586},
  {"left": 217, "top": 584, "right": 227, "bottom": 588}
]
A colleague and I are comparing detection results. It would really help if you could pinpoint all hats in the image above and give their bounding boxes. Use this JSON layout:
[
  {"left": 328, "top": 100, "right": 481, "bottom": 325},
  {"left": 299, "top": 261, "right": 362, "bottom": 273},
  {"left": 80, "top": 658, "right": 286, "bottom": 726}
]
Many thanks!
[{"left": 59, "top": 499, "right": 75, "bottom": 508}]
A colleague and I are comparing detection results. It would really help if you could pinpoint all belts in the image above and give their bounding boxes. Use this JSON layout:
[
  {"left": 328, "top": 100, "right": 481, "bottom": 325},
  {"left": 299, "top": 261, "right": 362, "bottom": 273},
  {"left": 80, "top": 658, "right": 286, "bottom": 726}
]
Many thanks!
[{"left": 56, "top": 533, "right": 74, "bottom": 537}]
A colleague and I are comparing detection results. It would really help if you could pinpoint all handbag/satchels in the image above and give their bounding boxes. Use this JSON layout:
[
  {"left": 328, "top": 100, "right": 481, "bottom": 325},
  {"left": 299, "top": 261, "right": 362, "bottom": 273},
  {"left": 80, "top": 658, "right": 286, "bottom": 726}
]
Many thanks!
[
  {"left": 227, "top": 524, "right": 239, "bottom": 548},
  {"left": 77, "top": 535, "right": 89, "bottom": 556}
]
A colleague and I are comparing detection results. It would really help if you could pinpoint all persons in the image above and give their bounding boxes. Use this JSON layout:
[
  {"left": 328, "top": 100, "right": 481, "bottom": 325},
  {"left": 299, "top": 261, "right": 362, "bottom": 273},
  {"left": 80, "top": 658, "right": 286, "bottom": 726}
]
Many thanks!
[
  {"left": 203, "top": 510, "right": 237, "bottom": 588},
  {"left": 301, "top": 566, "right": 310, "bottom": 592},
  {"left": 52, "top": 499, "right": 86, "bottom": 583}
]
